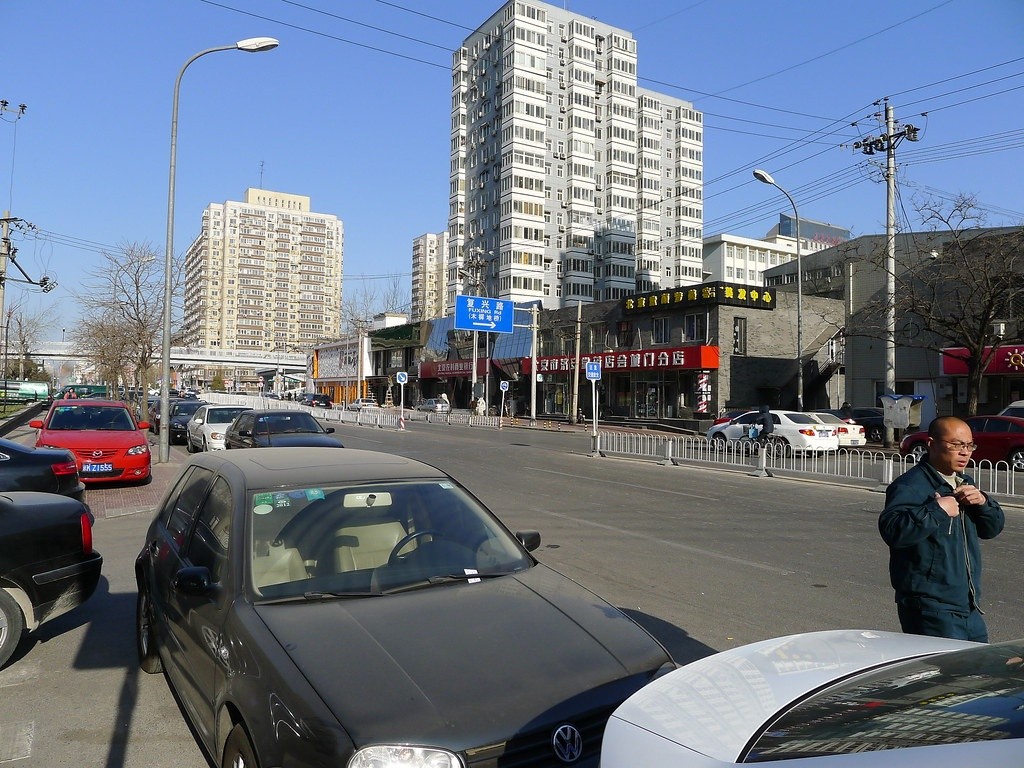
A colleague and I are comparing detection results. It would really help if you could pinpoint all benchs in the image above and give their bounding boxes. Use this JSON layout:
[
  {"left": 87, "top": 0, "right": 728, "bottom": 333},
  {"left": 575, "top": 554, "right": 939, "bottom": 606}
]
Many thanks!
[{"left": 275, "top": 507, "right": 344, "bottom": 562}]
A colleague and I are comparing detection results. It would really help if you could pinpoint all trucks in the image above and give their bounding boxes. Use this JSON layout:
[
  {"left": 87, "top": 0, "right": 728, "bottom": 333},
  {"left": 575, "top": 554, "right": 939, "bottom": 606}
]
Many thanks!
[
  {"left": 50, "top": 383, "right": 111, "bottom": 406},
  {"left": 0, "top": 378, "right": 53, "bottom": 407}
]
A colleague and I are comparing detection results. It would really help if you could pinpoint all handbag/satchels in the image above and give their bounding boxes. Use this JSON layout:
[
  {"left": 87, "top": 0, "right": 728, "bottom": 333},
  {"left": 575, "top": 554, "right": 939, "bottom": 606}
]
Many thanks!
[{"left": 748, "top": 424, "right": 758, "bottom": 438}]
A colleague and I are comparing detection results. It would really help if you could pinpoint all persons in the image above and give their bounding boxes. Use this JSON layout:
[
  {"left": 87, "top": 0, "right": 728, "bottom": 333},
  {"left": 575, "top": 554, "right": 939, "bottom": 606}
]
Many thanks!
[
  {"left": 64, "top": 388, "right": 77, "bottom": 399},
  {"left": 877, "top": 416, "right": 1006, "bottom": 642},
  {"left": 294, "top": 390, "right": 296, "bottom": 400},
  {"left": 437, "top": 392, "right": 450, "bottom": 404},
  {"left": 755, "top": 404, "right": 773, "bottom": 441},
  {"left": 840, "top": 402, "right": 853, "bottom": 418}
]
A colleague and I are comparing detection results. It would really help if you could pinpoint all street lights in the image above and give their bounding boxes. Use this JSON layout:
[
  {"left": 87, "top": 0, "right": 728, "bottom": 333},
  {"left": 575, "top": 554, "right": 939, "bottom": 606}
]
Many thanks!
[
  {"left": 326, "top": 307, "right": 349, "bottom": 411},
  {"left": 112, "top": 254, "right": 158, "bottom": 400},
  {"left": 458, "top": 269, "right": 489, "bottom": 419},
  {"left": 753, "top": 168, "right": 805, "bottom": 413},
  {"left": 62, "top": 328, "right": 65, "bottom": 342},
  {"left": 158, "top": 37, "right": 282, "bottom": 463}
]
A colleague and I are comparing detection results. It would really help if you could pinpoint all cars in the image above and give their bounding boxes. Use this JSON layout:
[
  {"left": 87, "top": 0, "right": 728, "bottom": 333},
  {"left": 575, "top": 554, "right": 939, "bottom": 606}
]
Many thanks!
[
  {"left": 223, "top": 408, "right": 344, "bottom": 449},
  {"left": 264, "top": 393, "right": 279, "bottom": 399},
  {"left": 596, "top": 627, "right": 1024, "bottom": 768},
  {"left": 117, "top": 385, "right": 208, "bottom": 446},
  {"left": 185, "top": 403, "right": 261, "bottom": 452},
  {"left": 82, "top": 391, "right": 110, "bottom": 399},
  {"left": 1, "top": 435, "right": 87, "bottom": 502},
  {"left": 707, "top": 410, "right": 840, "bottom": 459},
  {"left": 231, "top": 391, "right": 248, "bottom": 395},
  {"left": 417, "top": 397, "right": 450, "bottom": 415},
  {"left": 29, "top": 398, "right": 152, "bottom": 483},
  {"left": 295, "top": 392, "right": 334, "bottom": 409},
  {"left": 898, "top": 415, "right": 1024, "bottom": 474},
  {"left": 803, "top": 411, "right": 867, "bottom": 455},
  {"left": 0, "top": 488, "right": 104, "bottom": 673},
  {"left": 829, "top": 406, "right": 898, "bottom": 443},
  {"left": 347, "top": 398, "right": 378, "bottom": 412},
  {"left": 131, "top": 444, "right": 681, "bottom": 768}
]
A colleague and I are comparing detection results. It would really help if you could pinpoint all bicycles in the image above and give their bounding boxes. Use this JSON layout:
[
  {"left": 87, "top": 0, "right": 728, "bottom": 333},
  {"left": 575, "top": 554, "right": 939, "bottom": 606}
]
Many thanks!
[{"left": 735, "top": 419, "right": 784, "bottom": 460}]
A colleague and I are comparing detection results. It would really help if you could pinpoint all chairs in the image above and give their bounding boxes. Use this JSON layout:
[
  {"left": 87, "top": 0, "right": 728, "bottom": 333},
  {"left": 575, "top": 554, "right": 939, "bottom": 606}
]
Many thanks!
[
  {"left": 61, "top": 410, "right": 118, "bottom": 428},
  {"left": 250, "top": 523, "right": 308, "bottom": 589},
  {"left": 333, "top": 501, "right": 411, "bottom": 571}
]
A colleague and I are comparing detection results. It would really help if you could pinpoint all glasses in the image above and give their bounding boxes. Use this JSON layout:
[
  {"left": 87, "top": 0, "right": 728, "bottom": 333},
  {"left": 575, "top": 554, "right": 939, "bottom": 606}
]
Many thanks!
[{"left": 933, "top": 438, "right": 977, "bottom": 452}]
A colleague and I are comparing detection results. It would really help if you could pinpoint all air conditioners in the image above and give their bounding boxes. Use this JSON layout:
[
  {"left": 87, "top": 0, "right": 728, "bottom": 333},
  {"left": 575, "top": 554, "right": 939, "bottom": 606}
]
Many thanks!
[
  {"left": 598, "top": 209, "right": 603, "bottom": 214},
  {"left": 478, "top": 112, "right": 481, "bottom": 118},
  {"left": 492, "top": 130, "right": 495, "bottom": 135},
  {"left": 562, "top": 36, "right": 568, "bottom": 42},
  {"left": 482, "top": 205, "right": 486, "bottom": 211},
  {"left": 483, "top": 158, "right": 487, "bottom": 163},
  {"left": 494, "top": 175, "right": 498, "bottom": 179},
  {"left": 493, "top": 201, "right": 497, "bottom": 205},
  {"left": 545, "top": 263, "right": 550, "bottom": 270},
  {"left": 480, "top": 182, "right": 484, "bottom": 188},
  {"left": 562, "top": 201, "right": 568, "bottom": 206},
  {"left": 490, "top": 155, "right": 495, "bottom": 160},
  {"left": 560, "top": 225, "right": 565, "bottom": 232},
  {"left": 597, "top": 254, "right": 603, "bottom": 260},
  {"left": 492, "top": 272, "right": 496, "bottom": 277},
  {"left": 480, "top": 229, "right": 484, "bottom": 236},
  {"left": 480, "top": 137, "right": 485, "bottom": 143},
  {"left": 560, "top": 83, "right": 566, "bottom": 89},
  {"left": 596, "top": 116, "right": 602, "bottom": 121},
  {"left": 560, "top": 59, "right": 566, "bottom": 64},
  {"left": 561, "top": 153, "right": 567, "bottom": 158},
  {"left": 484, "top": 43, "right": 490, "bottom": 50},
  {"left": 596, "top": 185, "right": 602, "bottom": 190},
  {"left": 595, "top": 277, "right": 599, "bottom": 282},
  {"left": 495, "top": 81, "right": 500, "bottom": 87},
  {"left": 598, "top": 47, "right": 603, "bottom": 53},
  {"left": 561, "top": 106, "right": 566, "bottom": 112},
  {"left": 597, "top": 231, "right": 601, "bottom": 236},
  {"left": 589, "top": 249, "right": 594, "bottom": 254},
  {"left": 472, "top": 142, "right": 475, "bottom": 148},
  {"left": 473, "top": 54, "right": 478, "bottom": 60},
  {"left": 553, "top": 152, "right": 558, "bottom": 157},
  {"left": 480, "top": 70, "right": 485, "bottom": 76},
  {"left": 469, "top": 233, "right": 474, "bottom": 239},
  {"left": 662, "top": 196, "right": 664, "bottom": 200}
]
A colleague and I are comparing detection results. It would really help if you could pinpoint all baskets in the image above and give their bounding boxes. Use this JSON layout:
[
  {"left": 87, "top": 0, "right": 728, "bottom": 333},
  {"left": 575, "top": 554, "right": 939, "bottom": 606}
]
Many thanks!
[{"left": 743, "top": 425, "right": 752, "bottom": 434}]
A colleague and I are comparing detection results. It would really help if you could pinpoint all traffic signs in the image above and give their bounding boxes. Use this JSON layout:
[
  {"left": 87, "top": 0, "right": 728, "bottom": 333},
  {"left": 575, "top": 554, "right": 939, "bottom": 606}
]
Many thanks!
[{"left": 454, "top": 294, "right": 515, "bottom": 335}]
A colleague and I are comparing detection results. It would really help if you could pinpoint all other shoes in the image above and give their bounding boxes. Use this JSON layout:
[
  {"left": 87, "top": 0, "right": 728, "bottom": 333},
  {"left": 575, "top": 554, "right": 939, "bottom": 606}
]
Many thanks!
[{"left": 755, "top": 448, "right": 758, "bottom": 452}]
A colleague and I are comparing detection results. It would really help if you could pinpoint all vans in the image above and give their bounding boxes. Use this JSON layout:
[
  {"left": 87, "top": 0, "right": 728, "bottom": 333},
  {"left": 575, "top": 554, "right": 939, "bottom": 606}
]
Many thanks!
[{"left": 996, "top": 399, "right": 1024, "bottom": 418}]
